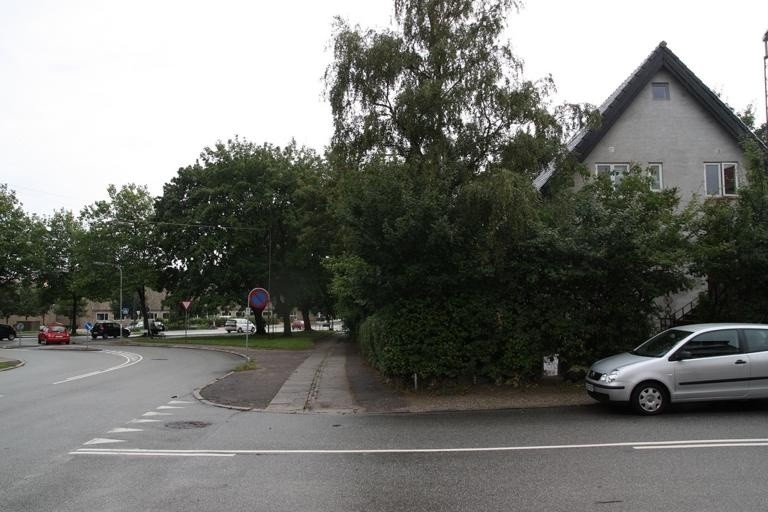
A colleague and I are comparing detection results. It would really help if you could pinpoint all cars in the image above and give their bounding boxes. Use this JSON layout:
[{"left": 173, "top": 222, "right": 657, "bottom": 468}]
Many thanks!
[
  {"left": 291, "top": 320, "right": 304, "bottom": 330},
  {"left": 0, "top": 323, "right": 16, "bottom": 342},
  {"left": 124, "top": 319, "right": 167, "bottom": 332},
  {"left": 37, "top": 322, "right": 71, "bottom": 346}
]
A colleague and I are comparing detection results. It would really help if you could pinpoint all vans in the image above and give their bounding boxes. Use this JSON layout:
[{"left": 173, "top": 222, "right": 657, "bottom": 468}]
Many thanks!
[
  {"left": 581, "top": 321, "right": 767, "bottom": 415},
  {"left": 224, "top": 319, "right": 256, "bottom": 332}
]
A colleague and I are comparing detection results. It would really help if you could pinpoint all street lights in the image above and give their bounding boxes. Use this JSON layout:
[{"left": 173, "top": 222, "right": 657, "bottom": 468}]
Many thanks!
[{"left": 92, "top": 262, "right": 123, "bottom": 343}]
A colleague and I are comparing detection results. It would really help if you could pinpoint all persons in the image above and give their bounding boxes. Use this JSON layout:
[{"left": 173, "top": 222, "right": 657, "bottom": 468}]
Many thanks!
[{"left": 149, "top": 320, "right": 155, "bottom": 338}]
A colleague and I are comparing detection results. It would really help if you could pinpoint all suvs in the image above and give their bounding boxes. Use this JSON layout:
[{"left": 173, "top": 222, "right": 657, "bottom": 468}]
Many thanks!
[{"left": 90, "top": 322, "right": 131, "bottom": 338}]
